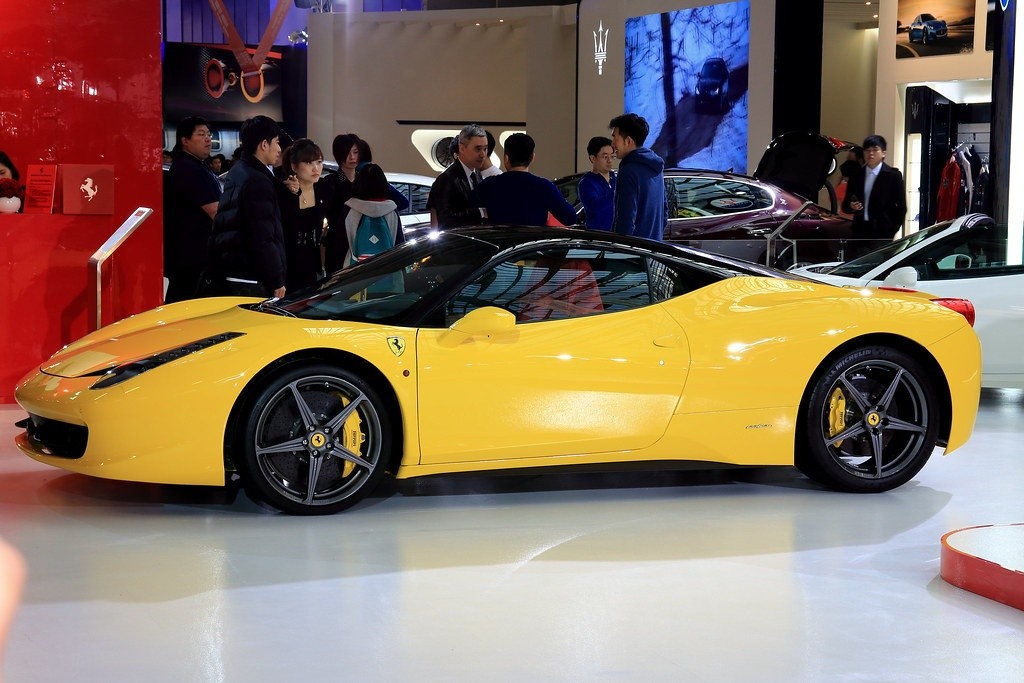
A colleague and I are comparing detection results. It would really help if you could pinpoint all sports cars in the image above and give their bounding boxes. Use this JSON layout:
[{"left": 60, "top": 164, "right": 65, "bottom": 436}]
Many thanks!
[{"left": 11, "top": 222, "right": 982, "bottom": 514}]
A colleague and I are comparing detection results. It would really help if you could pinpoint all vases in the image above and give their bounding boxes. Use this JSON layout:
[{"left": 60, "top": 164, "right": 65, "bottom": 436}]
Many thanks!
[{"left": 0, "top": 196, "right": 21, "bottom": 213}]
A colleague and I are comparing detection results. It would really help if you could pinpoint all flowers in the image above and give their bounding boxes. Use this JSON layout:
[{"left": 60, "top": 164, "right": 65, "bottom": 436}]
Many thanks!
[{"left": 0, "top": 177, "right": 26, "bottom": 199}]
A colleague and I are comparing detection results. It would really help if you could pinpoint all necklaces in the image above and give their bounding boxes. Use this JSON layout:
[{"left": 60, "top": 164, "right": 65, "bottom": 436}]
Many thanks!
[{"left": 302, "top": 193, "right": 308, "bottom": 204}]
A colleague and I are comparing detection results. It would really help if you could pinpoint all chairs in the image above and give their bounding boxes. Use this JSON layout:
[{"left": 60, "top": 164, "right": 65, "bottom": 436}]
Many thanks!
[{"left": 520, "top": 259, "right": 603, "bottom": 322}]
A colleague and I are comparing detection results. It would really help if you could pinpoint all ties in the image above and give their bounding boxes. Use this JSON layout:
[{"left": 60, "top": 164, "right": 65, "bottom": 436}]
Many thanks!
[{"left": 471, "top": 172, "right": 479, "bottom": 188}]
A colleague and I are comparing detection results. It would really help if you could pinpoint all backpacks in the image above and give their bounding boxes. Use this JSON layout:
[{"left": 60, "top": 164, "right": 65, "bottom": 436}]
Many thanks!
[{"left": 350, "top": 215, "right": 394, "bottom": 270}]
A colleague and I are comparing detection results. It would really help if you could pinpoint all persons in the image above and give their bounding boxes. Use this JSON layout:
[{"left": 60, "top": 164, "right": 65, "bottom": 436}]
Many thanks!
[
  {"left": 163, "top": 116, "right": 222, "bottom": 304},
  {"left": 212, "top": 115, "right": 409, "bottom": 303},
  {"left": 163, "top": 147, "right": 241, "bottom": 176},
  {"left": 0, "top": 151, "right": 26, "bottom": 213},
  {"left": 834, "top": 136, "right": 907, "bottom": 261},
  {"left": 427, "top": 114, "right": 668, "bottom": 241}
]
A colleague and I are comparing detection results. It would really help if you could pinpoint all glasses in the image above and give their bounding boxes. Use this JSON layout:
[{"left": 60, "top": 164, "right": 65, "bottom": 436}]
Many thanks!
[
  {"left": 594, "top": 154, "right": 617, "bottom": 161},
  {"left": 192, "top": 132, "right": 214, "bottom": 139}
]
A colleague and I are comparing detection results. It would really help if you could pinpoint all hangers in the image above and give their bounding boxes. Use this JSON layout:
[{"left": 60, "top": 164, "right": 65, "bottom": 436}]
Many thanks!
[
  {"left": 950, "top": 138, "right": 972, "bottom": 163},
  {"left": 979, "top": 156, "right": 989, "bottom": 175}
]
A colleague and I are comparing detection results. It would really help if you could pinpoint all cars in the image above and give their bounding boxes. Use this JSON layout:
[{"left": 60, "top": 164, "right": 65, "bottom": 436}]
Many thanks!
[
  {"left": 215, "top": 160, "right": 435, "bottom": 243},
  {"left": 546, "top": 131, "right": 1024, "bottom": 389}
]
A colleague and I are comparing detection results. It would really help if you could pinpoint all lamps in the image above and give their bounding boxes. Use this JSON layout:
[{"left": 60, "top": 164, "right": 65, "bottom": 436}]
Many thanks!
[
  {"left": 288, "top": 28, "right": 308, "bottom": 46},
  {"left": 905, "top": 133, "right": 922, "bottom": 237}
]
